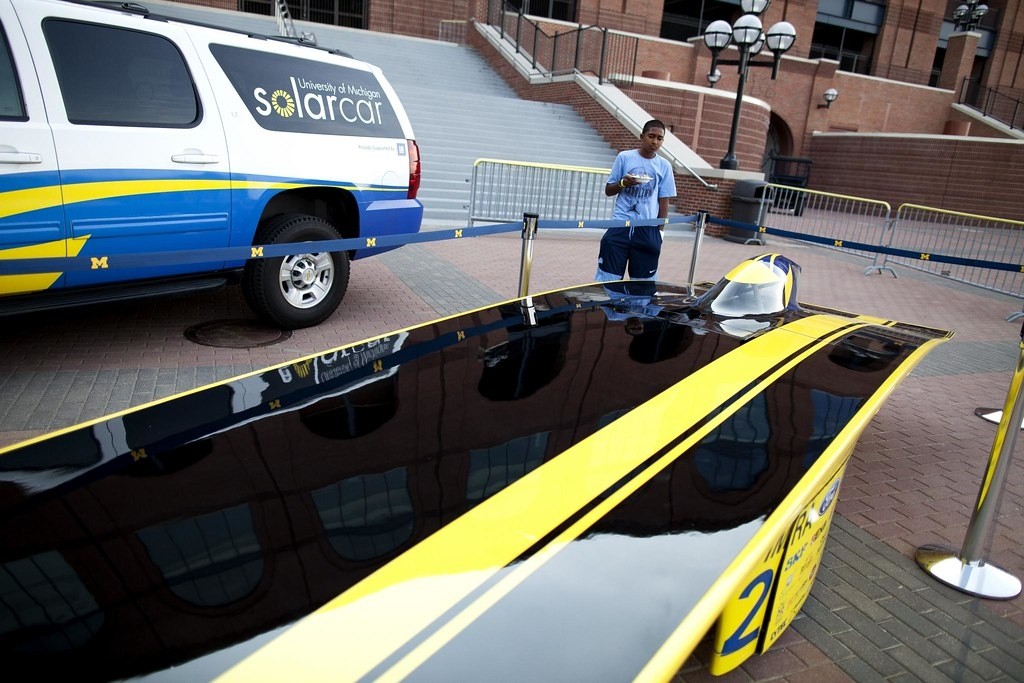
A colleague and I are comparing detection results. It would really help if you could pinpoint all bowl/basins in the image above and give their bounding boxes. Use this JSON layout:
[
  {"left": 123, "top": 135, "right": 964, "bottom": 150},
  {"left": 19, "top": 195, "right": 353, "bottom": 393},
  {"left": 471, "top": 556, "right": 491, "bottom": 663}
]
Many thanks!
[{"left": 632, "top": 176, "right": 654, "bottom": 184}]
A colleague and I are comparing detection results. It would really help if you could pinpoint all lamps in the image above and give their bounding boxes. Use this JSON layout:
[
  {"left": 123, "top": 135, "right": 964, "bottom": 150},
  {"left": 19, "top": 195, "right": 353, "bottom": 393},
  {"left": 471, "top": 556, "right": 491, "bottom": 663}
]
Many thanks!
[
  {"left": 818, "top": 89, "right": 838, "bottom": 108},
  {"left": 706, "top": 69, "right": 721, "bottom": 88}
]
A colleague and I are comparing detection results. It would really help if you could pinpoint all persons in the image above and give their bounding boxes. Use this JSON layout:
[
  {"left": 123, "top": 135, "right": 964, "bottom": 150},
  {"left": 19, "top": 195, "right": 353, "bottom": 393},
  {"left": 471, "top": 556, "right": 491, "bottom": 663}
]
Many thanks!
[
  {"left": 599, "top": 281, "right": 664, "bottom": 335},
  {"left": 595, "top": 119, "right": 678, "bottom": 283}
]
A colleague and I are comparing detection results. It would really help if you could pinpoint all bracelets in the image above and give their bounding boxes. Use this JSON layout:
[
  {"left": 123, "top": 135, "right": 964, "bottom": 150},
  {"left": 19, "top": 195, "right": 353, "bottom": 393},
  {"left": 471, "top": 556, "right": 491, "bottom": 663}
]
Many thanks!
[
  {"left": 659, "top": 230, "right": 664, "bottom": 238},
  {"left": 617, "top": 179, "right": 625, "bottom": 188}
]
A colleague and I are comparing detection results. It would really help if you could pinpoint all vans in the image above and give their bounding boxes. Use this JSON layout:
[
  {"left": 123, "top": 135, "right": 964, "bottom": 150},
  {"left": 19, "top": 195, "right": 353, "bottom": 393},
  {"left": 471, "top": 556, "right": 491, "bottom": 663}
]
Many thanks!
[{"left": 0, "top": 0, "right": 422, "bottom": 330}]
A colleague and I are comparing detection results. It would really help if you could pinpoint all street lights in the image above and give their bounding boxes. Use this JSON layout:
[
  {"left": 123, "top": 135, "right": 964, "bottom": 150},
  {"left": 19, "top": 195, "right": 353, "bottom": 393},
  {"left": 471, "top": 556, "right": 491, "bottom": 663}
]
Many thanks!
[{"left": 703, "top": 1, "right": 797, "bottom": 170}]
